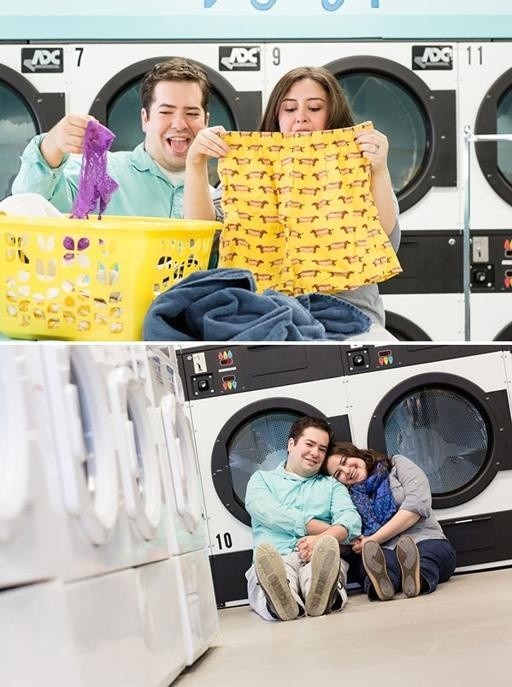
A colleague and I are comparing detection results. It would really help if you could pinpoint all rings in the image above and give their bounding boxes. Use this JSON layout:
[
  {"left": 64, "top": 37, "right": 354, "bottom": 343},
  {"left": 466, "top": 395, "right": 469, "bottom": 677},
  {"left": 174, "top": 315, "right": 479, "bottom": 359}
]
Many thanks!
[{"left": 375, "top": 143, "right": 380, "bottom": 153}]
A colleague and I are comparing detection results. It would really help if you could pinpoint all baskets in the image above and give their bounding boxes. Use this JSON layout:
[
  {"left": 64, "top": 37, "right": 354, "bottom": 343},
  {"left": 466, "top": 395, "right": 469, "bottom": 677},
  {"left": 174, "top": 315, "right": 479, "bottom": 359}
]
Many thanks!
[{"left": 1, "top": 213, "right": 224, "bottom": 344}]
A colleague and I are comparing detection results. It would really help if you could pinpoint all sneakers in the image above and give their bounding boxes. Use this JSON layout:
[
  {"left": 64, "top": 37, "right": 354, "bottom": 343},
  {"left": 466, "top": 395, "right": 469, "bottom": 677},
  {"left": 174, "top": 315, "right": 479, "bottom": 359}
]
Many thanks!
[
  {"left": 253, "top": 542, "right": 299, "bottom": 621},
  {"left": 396, "top": 535, "right": 420, "bottom": 597},
  {"left": 304, "top": 535, "right": 341, "bottom": 617},
  {"left": 361, "top": 540, "right": 395, "bottom": 602}
]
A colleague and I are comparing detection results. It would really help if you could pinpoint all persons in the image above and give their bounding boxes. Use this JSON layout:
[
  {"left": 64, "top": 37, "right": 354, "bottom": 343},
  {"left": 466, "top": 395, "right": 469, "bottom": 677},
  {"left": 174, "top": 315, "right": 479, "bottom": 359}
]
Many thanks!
[
  {"left": 0, "top": 55, "right": 221, "bottom": 270},
  {"left": 293, "top": 440, "right": 457, "bottom": 602},
  {"left": 243, "top": 415, "right": 363, "bottom": 622},
  {"left": 182, "top": 64, "right": 403, "bottom": 331}
]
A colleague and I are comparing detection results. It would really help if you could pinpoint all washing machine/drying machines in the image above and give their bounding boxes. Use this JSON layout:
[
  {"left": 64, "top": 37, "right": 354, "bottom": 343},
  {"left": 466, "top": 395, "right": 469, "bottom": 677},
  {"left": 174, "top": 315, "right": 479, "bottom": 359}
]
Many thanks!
[
  {"left": 0, "top": 345, "right": 512, "bottom": 687},
  {"left": 0, "top": 42, "right": 512, "bottom": 341}
]
